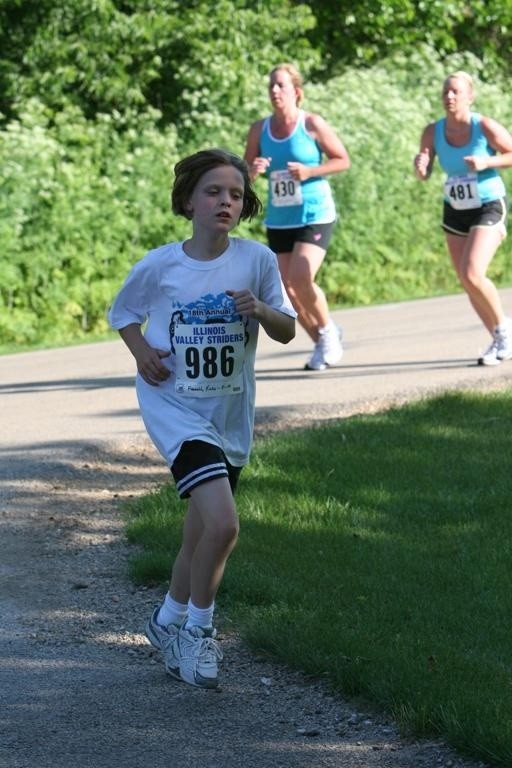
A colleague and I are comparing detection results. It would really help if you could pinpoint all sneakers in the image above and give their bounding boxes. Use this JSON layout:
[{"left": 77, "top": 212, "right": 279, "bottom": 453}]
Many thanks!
[
  {"left": 305, "top": 325, "right": 342, "bottom": 369},
  {"left": 145, "top": 607, "right": 217, "bottom": 689},
  {"left": 478, "top": 318, "right": 512, "bottom": 365}
]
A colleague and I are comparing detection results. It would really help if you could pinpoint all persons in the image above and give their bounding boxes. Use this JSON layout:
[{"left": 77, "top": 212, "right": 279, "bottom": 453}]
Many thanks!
[
  {"left": 414, "top": 72, "right": 511, "bottom": 364},
  {"left": 244, "top": 64, "right": 350, "bottom": 370},
  {"left": 107, "top": 148, "right": 297, "bottom": 690}
]
